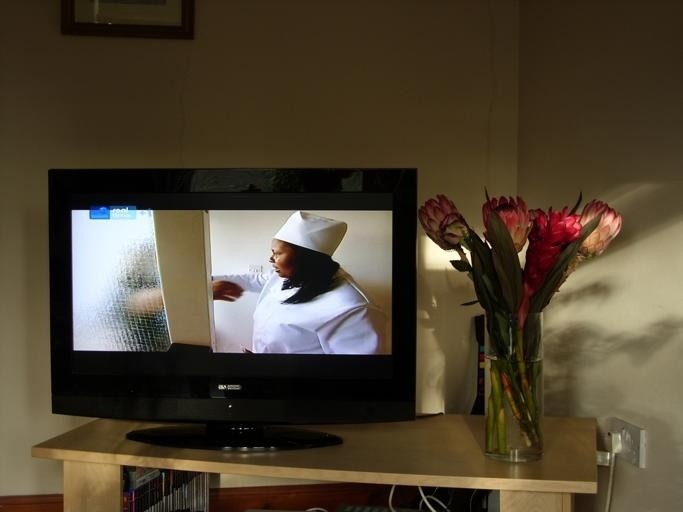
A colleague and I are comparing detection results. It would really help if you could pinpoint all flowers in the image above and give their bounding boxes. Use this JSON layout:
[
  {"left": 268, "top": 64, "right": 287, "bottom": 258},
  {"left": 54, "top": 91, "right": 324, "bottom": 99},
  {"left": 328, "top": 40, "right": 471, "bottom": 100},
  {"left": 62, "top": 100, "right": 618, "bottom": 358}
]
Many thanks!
[{"left": 416, "top": 185, "right": 624, "bottom": 454}]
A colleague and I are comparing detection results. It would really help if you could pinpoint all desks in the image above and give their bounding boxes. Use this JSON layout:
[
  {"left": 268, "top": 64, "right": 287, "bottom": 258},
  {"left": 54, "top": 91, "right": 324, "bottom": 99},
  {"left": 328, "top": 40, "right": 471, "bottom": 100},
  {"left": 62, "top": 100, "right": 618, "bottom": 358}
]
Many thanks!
[{"left": 26, "top": 416, "right": 600, "bottom": 511}]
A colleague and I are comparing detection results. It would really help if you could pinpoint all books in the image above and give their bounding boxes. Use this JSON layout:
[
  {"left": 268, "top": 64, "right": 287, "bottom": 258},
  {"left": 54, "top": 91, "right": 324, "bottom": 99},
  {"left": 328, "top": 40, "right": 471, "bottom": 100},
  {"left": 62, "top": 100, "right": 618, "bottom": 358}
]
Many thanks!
[{"left": 121, "top": 466, "right": 209, "bottom": 511}]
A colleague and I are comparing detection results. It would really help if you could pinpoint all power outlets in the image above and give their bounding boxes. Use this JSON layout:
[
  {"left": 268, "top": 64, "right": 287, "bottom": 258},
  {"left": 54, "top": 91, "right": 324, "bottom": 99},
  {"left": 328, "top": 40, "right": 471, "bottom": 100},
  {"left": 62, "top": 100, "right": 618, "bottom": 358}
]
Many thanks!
[{"left": 607, "top": 415, "right": 646, "bottom": 470}]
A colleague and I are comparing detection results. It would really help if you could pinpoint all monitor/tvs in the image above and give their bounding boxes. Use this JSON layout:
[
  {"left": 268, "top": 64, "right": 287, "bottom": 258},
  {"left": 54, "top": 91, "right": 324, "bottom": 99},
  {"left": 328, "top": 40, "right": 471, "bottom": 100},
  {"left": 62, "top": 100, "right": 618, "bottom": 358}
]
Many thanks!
[{"left": 46, "top": 167, "right": 419, "bottom": 452}]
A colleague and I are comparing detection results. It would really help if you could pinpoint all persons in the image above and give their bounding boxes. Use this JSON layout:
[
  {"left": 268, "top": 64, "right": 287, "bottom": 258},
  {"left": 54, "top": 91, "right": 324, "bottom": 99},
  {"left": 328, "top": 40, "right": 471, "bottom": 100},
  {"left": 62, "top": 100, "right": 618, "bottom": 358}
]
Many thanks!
[
  {"left": 210, "top": 210, "right": 379, "bottom": 354},
  {"left": 99, "top": 236, "right": 245, "bottom": 352}
]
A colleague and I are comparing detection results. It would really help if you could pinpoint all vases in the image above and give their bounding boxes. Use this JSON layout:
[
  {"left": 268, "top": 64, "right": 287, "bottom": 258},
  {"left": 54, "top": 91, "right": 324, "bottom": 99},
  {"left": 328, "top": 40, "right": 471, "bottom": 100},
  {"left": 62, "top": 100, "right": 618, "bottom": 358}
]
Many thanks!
[{"left": 482, "top": 310, "right": 546, "bottom": 464}]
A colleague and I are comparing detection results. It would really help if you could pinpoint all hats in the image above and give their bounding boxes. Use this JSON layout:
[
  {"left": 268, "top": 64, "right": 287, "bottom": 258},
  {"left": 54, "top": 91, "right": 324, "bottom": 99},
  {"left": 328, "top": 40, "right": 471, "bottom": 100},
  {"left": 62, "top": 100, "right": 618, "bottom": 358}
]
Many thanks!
[{"left": 274, "top": 211, "right": 348, "bottom": 256}]
